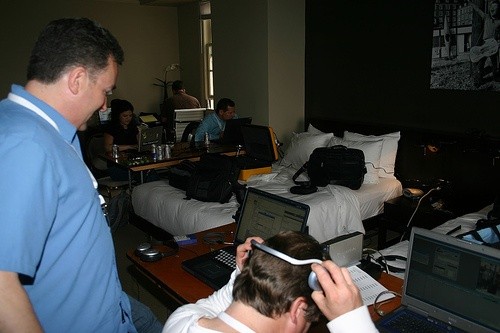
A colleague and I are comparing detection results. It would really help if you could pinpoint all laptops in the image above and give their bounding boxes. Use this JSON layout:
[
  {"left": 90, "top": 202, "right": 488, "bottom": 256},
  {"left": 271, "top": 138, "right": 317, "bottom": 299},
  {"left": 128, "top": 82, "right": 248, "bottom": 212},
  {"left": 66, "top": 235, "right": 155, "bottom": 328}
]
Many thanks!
[
  {"left": 99, "top": 107, "right": 112, "bottom": 124},
  {"left": 181, "top": 186, "right": 310, "bottom": 291},
  {"left": 210, "top": 116, "right": 252, "bottom": 146},
  {"left": 137, "top": 125, "right": 164, "bottom": 153},
  {"left": 374, "top": 227, "right": 500, "bottom": 333}
]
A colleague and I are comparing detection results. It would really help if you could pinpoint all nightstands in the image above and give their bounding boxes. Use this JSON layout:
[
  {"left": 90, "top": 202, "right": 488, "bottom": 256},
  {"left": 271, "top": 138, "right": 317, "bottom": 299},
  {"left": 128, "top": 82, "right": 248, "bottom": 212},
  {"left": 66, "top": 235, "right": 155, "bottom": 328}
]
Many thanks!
[{"left": 363, "top": 196, "right": 469, "bottom": 251}]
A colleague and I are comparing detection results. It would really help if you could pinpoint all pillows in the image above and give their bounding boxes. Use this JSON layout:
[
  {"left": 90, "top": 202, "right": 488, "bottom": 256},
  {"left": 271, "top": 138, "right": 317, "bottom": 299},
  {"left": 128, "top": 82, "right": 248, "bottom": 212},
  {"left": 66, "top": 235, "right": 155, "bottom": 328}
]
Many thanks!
[
  {"left": 280, "top": 129, "right": 334, "bottom": 170},
  {"left": 308, "top": 124, "right": 340, "bottom": 146},
  {"left": 342, "top": 130, "right": 401, "bottom": 179},
  {"left": 328, "top": 137, "right": 383, "bottom": 184}
]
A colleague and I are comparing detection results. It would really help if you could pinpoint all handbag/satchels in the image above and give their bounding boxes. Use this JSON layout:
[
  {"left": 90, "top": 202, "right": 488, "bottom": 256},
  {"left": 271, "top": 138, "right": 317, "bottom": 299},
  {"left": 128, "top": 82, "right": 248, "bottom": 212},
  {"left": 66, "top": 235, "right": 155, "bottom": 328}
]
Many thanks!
[
  {"left": 186, "top": 162, "right": 233, "bottom": 204},
  {"left": 308, "top": 144, "right": 367, "bottom": 190}
]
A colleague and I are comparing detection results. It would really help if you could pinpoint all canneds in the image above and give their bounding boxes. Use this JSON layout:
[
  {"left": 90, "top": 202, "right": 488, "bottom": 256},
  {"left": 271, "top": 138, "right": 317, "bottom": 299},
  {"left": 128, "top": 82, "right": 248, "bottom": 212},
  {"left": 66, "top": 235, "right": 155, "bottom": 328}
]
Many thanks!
[
  {"left": 152, "top": 145, "right": 171, "bottom": 160},
  {"left": 112, "top": 144, "right": 120, "bottom": 159},
  {"left": 204, "top": 132, "right": 211, "bottom": 145}
]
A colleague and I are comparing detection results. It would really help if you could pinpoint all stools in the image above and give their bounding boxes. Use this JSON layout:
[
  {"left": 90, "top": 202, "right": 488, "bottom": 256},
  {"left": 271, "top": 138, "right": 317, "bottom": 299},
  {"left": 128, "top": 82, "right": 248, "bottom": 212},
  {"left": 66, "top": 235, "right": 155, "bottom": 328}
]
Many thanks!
[{"left": 97, "top": 176, "right": 138, "bottom": 201}]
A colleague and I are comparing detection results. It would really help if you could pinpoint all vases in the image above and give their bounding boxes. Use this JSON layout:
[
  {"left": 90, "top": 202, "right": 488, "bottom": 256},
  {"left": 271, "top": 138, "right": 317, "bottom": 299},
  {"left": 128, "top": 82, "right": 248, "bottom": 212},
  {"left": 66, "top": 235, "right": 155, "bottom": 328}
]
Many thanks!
[{"left": 159, "top": 103, "right": 170, "bottom": 114}]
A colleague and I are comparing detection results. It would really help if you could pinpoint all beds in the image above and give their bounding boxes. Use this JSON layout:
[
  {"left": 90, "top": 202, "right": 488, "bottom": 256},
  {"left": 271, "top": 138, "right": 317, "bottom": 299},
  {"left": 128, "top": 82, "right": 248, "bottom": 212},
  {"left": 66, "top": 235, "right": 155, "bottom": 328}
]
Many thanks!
[{"left": 127, "top": 118, "right": 433, "bottom": 238}]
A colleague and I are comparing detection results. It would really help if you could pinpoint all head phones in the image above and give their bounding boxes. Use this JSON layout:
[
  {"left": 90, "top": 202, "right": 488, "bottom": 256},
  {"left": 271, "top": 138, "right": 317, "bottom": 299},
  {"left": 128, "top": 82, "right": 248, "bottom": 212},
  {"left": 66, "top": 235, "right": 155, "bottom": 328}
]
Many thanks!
[
  {"left": 251, "top": 237, "right": 329, "bottom": 291},
  {"left": 134, "top": 240, "right": 180, "bottom": 263}
]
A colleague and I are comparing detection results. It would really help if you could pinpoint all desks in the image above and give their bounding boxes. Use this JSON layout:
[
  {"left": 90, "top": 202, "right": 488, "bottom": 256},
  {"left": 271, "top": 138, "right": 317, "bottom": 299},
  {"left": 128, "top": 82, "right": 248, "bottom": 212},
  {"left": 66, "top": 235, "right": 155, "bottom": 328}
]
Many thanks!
[
  {"left": 94, "top": 140, "right": 245, "bottom": 190},
  {"left": 129, "top": 223, "right": 404, "bottom": 333}
]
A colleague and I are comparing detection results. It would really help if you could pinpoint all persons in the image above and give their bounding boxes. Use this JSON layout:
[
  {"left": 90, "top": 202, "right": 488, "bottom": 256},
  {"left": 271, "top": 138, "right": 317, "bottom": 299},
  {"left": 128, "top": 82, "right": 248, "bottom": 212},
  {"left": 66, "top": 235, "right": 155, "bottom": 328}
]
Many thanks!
[
  {"left": 104, "top": 99, "right": 140, "bottom": 178},
  {"left": 161, "top": 230, "right": 379, "bottom": 333},
  {"left": 0, "top": 16, "right": 164, "bottom": 333},
  {"left": 194, "top": 98, "right": 235, "bottom": 141},
  {"left": 162, "top": 80, "right": 201, "bottom": 129}
]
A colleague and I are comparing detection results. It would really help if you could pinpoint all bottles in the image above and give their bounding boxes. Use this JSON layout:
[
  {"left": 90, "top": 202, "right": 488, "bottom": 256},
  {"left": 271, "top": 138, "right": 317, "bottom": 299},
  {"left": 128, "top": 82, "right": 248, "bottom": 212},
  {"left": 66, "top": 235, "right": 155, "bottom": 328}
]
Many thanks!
[{"left": 204, "top": 132, "right": 210, "bottom": 150}]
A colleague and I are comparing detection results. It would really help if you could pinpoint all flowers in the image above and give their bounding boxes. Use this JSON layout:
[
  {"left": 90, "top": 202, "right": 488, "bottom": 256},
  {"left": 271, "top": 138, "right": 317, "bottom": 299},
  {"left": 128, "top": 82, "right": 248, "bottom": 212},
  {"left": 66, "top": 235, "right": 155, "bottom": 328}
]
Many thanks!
[{"left": 152, "top": 64, "right": 184, "bottom": 103}]
